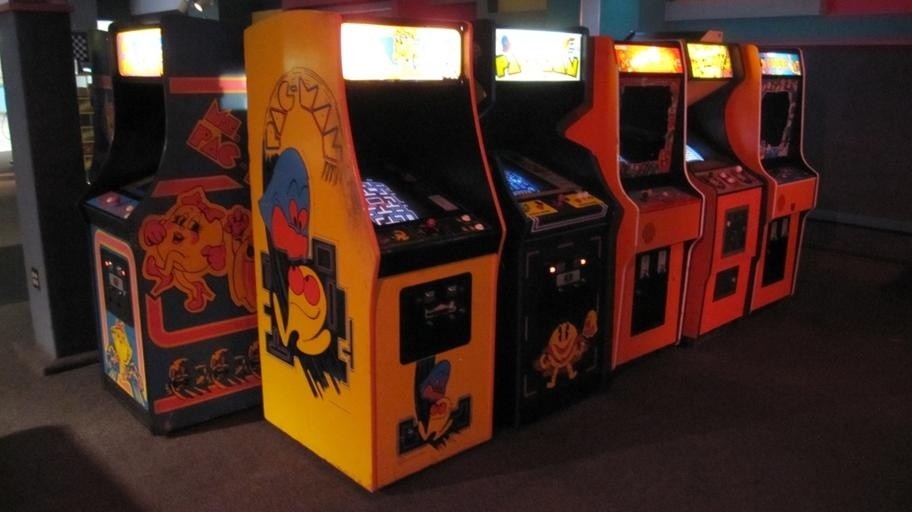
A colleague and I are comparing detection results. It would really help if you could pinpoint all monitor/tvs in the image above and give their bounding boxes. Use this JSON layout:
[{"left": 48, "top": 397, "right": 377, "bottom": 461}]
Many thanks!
[
  {"left": 685, "top": 144, "right": 705, "bottom": 163},
  {"left": 360, "top": 179, "right": 420, "bottom": 226},
  {"left": 499, "top": 165, "right": 539, "bottom": 196}
]
